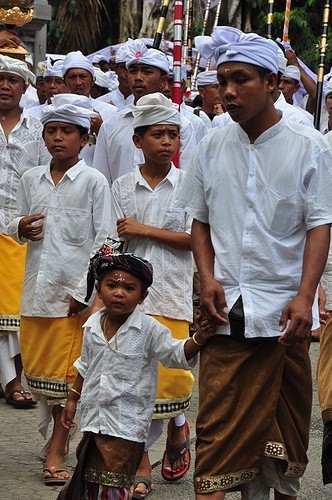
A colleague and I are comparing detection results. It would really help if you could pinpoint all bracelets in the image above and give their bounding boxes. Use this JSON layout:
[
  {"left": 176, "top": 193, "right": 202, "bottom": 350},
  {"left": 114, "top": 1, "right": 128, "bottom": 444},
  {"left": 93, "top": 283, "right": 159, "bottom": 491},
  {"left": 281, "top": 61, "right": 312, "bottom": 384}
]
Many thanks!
[
  {"left": 70, "top": 389, "right": 82, "bottom": 397},
  {"left": 192, "top": 333, "right": 205, "bottom": 347}
]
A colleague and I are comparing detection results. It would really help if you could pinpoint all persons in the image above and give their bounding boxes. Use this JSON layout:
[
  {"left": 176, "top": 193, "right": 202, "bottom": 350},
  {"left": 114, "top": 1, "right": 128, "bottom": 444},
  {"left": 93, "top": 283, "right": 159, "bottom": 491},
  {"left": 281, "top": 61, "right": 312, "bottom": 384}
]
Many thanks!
[{"left": 0, "top": 26, "right": 332, "bottom": 500}]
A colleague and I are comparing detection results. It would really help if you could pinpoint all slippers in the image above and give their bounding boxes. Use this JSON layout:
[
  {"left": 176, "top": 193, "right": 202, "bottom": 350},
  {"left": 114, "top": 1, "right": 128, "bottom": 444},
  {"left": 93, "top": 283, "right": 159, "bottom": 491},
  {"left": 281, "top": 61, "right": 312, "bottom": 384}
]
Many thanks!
[
  {"left": 4, "top": 390, "right": 38, "bottom": 406},
  {"left": 132, "top": 476, "right": 155, "bottom": 500},
  {"left": 42, "top": 468, "right": 69, "bottom": 484},
  {"left": 162, "top": 417, "right": 190, "bottom": 480}
]
